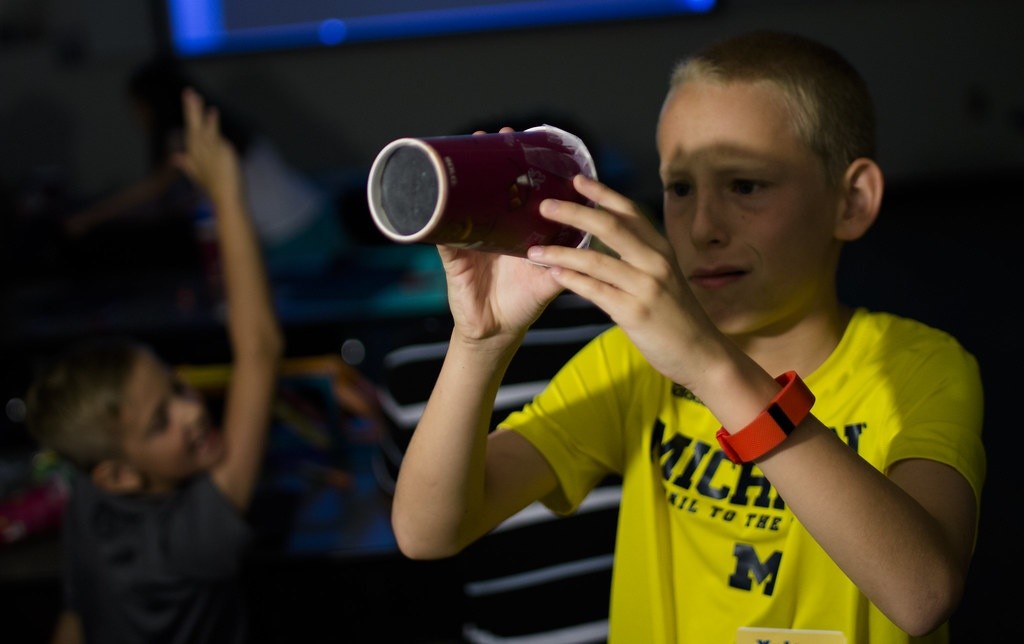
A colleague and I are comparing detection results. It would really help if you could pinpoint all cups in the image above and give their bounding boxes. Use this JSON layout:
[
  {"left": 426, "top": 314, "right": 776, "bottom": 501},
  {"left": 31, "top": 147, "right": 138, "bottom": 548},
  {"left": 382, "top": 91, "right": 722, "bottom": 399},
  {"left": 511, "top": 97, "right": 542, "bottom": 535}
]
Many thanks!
[{"left": 366, "top": 124, "right": 598, "bottom": 269}]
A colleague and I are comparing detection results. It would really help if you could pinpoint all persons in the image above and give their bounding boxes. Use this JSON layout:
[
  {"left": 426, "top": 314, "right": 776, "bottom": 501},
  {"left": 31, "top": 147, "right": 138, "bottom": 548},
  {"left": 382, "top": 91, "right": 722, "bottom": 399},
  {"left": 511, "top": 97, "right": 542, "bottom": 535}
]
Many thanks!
[
  {"left": 0, "top": 50, "right": 473, "bottom": 644},
  {"left": 389, "top": 30, "right": 987, "bottom": 643}
]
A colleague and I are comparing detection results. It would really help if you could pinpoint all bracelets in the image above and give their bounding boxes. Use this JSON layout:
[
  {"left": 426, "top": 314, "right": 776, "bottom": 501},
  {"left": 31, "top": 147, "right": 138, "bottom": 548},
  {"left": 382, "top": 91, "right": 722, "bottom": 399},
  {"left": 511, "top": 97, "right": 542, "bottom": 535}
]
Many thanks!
[{"left": 716, "top": 370, "right": 816, "bottom": 462}]
